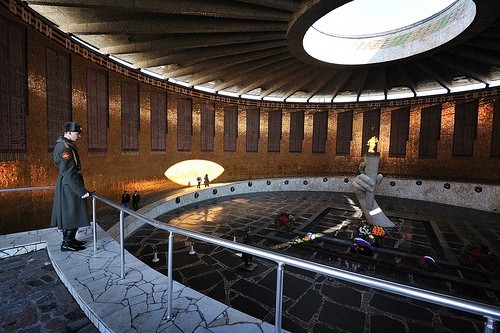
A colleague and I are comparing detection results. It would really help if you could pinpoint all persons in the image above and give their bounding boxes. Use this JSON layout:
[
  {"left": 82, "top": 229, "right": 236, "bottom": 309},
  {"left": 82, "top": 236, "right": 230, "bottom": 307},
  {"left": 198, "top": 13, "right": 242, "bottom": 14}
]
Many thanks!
[
  {"left": 50, "top": 122, "right": 91, "bottom": 252},
  {"left": 352, "top": 162, "right": 396, "bottom": 227},
  {"left": 203, "top": 174, "right": 210, "bottom": 187},
  {"left": 197, "top": 175, "right": 202, "bottom": 188},
  {"left": 121, "top": 191, "right": 141, "bottom": 214}
]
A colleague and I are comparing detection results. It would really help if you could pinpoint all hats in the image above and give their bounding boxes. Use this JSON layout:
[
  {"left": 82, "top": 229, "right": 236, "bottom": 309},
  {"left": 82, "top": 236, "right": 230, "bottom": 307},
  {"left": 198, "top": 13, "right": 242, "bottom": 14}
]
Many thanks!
[{"left": 62, "top": 122, "right": 83, "bottom": 132}]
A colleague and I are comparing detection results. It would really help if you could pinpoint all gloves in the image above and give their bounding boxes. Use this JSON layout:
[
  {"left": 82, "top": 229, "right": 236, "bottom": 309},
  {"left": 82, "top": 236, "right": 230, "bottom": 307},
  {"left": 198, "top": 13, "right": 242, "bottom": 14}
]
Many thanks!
[{"left": 81, "top": 192, "right": 90, "bottom": 199}]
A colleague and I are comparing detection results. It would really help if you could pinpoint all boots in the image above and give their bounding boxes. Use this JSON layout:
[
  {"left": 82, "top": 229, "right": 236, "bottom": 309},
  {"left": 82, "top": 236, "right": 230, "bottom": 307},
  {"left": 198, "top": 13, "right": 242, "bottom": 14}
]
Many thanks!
[
  {"left": 61, "top": 229, "right": 86, "bottom": 251},
  {"left": 63, "top": 229, "right": 87, "bottom": 245}
]
